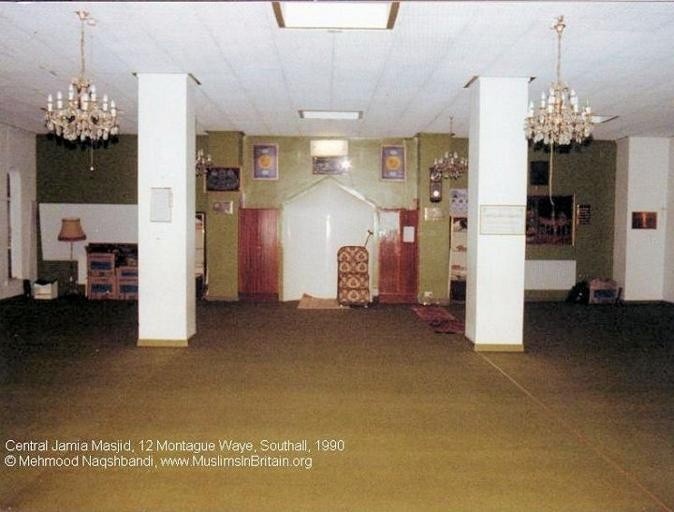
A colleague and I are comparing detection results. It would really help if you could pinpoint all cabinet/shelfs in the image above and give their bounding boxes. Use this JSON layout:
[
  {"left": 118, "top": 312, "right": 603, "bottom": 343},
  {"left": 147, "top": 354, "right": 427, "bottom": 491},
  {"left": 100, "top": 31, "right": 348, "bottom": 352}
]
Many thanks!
[{"left": 88, "top": 253, "right": 138, "bottom": 300}]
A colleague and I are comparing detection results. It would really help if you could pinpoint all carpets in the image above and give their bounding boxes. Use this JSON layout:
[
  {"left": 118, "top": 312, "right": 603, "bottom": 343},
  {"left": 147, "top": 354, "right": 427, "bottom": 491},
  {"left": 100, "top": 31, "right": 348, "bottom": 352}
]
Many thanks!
[
  {"left": 296, "top": 293, "right": 350, "bottom": 310},
  {"left": 411, "top": 304, "right": 464, "bottom": 335}
]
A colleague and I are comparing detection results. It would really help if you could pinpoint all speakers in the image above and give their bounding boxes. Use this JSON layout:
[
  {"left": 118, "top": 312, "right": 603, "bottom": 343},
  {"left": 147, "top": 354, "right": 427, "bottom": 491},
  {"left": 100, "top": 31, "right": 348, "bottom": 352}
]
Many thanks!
[{"left": 531, "top": 161, "right": 549, "bottom": 186}]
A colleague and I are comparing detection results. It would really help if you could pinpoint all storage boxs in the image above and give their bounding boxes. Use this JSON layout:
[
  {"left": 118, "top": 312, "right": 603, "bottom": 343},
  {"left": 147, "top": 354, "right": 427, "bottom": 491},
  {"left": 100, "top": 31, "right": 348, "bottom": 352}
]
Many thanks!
[{"left": 33, "top": 281, "right": 57, "bottom": 300}]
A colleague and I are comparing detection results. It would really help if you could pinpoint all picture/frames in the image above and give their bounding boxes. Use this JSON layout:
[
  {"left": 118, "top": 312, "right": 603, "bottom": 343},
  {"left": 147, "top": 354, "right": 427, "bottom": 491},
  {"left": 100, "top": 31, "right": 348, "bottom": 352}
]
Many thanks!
[
  {"left": 252, "top": 142, "right": 278, "bottom": 181},
  {"left": 379, "top": 144, "right": 407, "bottom": 182}
]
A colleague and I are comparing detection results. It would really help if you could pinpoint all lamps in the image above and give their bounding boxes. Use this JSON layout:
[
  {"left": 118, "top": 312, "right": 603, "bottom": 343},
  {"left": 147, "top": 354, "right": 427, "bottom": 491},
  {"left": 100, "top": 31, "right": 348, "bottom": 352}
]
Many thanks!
[
  {"left": 40, "top": 10, "right": 120, "bottom": 171},
  {"left": 58, "top": 217, "right": 86, "bottom": 293},
  {"left": 524, "top": 16, "right": 596, "bottom": 147},
  {"left": 429, "top": 113, "right": 469, "bottom": 182},
  {"left": 196, "top": 149, "right": 213, "bottom": 176}
]
